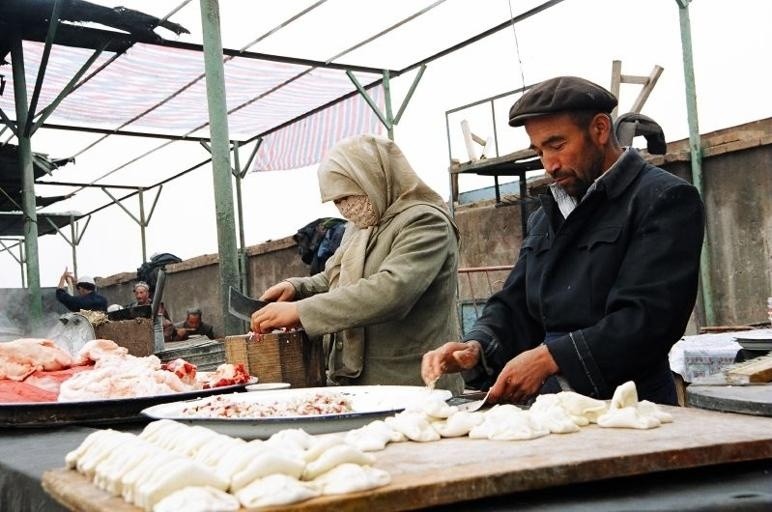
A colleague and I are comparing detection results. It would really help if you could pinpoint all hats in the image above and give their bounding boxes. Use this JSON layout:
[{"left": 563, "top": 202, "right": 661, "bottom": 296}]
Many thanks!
[
  {"left": 76, "top": 276, "right": 96, "bottom": 284},
  {"left": 509, "top": 77, "right": 617, "bottom": 126},
  {"left": 187, "top": 309, "right": 202, "bottom": 314},
  {"left": 134, "top": 281, "right": 149, "bottom": 291}
]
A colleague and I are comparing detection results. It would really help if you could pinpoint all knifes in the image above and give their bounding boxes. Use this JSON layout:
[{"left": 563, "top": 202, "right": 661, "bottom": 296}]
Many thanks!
[{"left": 228, "top": 284, "right": 272, "bottom": 325}]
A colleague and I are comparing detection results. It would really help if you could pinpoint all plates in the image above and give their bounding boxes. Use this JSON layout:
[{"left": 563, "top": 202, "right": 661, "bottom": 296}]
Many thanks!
[
  {"left": 139, "top": 385, "right": 453, "bottom": 440},
  {"left": 0, "top": 369, "right": 258, "bottom": 429},
  {"left": 732, "top": 331, "right": 772, "bottom": 351}
]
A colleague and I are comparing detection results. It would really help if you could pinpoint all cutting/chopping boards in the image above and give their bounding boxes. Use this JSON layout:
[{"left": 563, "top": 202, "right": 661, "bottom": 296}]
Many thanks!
[
  {"left": 224, "top": 329, "right": 308, "bottom": 389},
  {"left": 42, "top": 394, "right": 772, "bottom": 512}
]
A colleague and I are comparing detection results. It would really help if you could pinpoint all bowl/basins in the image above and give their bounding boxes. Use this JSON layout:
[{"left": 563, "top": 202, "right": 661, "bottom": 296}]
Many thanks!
[{"left": 246, "top": 383, "right": 291, "bottom": 394}]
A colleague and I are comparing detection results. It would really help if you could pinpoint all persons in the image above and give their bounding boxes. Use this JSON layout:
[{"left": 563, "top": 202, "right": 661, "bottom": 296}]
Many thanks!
[
  {"left": 421, "top": 76, "right": 707, "bottom": 412},
  {"left": 122, "top": 280, "right": 154, "bottom": 309},
  {"left": 242, "top": 131, "right": 467, "bottom": 395},
  {"left": 172, "top": 308, "right": 214, "bottom": 342},
  {"left": 55, "top": 266, "right": 107, "bottom": 315}
]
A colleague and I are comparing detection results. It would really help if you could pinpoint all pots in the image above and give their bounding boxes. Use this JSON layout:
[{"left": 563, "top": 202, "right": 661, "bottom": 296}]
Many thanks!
[{"left": 107, "top": 304, "right": 152, "bottom": 321}]
